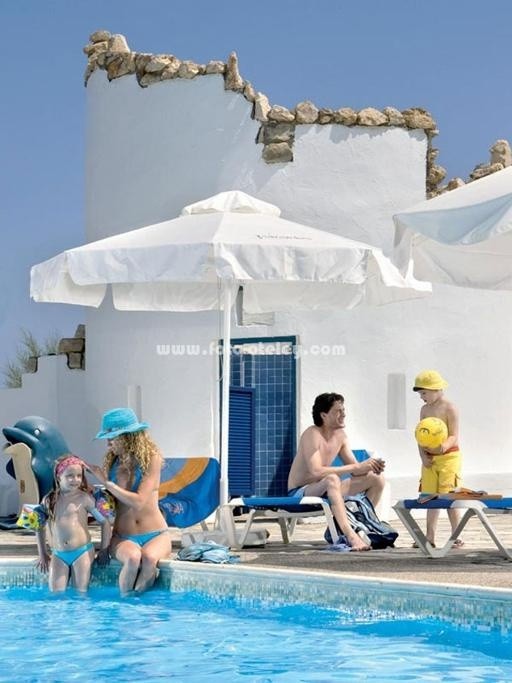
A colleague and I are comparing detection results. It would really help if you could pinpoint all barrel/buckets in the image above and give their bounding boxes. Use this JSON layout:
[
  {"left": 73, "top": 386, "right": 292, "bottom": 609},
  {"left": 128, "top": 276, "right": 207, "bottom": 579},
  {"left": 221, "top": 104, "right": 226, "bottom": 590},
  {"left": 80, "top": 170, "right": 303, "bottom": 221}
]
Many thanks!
[
  {"left": 450, "top": 538, "right": 465, "bottom": 548},
  {"left": 412, "top": 539, "right": 436, "bottom": 548}
]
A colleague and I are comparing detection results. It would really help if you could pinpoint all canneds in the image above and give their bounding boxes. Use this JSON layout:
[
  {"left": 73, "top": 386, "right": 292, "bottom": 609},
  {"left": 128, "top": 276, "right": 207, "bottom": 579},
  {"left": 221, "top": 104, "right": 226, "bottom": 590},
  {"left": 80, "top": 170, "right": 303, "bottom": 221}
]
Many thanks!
[
  {"left": 17, "top": 504, "right": 48, "bottom": 528},
  {"left": 95, "top": 491, "right": 115, "bottom": 516}
]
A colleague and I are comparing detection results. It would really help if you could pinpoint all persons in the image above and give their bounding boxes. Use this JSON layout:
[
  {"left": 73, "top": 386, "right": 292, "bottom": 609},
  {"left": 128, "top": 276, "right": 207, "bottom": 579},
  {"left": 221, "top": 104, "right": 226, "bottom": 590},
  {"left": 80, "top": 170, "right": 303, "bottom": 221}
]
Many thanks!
[
  {"left": 33, "top": 454, "right": 112, "bottom": 593},
  {"left": 412, "top": 371, "right": 463, "bottom": 548},
  {"left": 80, "top": 408, "right": 171, "bottom": 592},
  {"left": 288, "top": 393, "right": 385, "bottom": 551}
]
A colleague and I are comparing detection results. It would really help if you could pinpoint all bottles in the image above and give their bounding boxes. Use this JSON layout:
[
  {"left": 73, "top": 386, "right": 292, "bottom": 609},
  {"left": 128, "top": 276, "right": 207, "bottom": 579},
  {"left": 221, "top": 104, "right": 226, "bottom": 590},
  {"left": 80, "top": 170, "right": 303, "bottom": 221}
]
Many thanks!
[
  {"left": 96, "top": 407, "right": 150, "bottom": 440},
  {"left": 413, "top": 369, "right": 449, "bottom": 392}
]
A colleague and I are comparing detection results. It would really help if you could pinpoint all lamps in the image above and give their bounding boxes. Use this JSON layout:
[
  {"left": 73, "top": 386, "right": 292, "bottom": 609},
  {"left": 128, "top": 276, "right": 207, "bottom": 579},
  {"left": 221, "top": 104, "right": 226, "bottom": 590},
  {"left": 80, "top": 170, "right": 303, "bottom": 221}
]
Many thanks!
[
  {"left": 88, "top": 457, "right": 220, "bottom": 544},
  {"left": 390, "top": 496, "right": 512, "bottom": 562},
  {"left": 220, "top": 449, "right": 370, "bottom": 550}
]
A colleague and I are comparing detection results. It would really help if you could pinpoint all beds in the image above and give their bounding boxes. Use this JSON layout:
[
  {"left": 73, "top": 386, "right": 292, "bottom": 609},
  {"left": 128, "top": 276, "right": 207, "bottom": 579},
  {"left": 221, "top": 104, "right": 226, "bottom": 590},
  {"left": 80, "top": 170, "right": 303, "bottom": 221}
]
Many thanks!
[
  {"left": 30, "top": 188, "right": 433, "bottom": 535},
  {"left": 392, "top": 162, "right": 512, "bottom": 292}
]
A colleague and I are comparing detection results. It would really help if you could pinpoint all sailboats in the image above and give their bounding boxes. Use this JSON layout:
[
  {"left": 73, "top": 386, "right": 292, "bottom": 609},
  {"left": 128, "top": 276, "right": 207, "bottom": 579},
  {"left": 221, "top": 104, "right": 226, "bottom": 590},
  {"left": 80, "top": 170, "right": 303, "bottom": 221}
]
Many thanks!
[{"left": 415, "top": 418, "right": 447, "bottom": 448}]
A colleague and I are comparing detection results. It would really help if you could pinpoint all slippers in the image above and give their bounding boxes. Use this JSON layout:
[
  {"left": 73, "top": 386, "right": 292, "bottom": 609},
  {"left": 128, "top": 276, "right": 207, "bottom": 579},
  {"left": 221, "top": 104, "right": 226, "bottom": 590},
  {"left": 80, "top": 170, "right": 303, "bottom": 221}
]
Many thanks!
[{"left": 324, "top": 495, "right": 399, "bottom": 549}]
